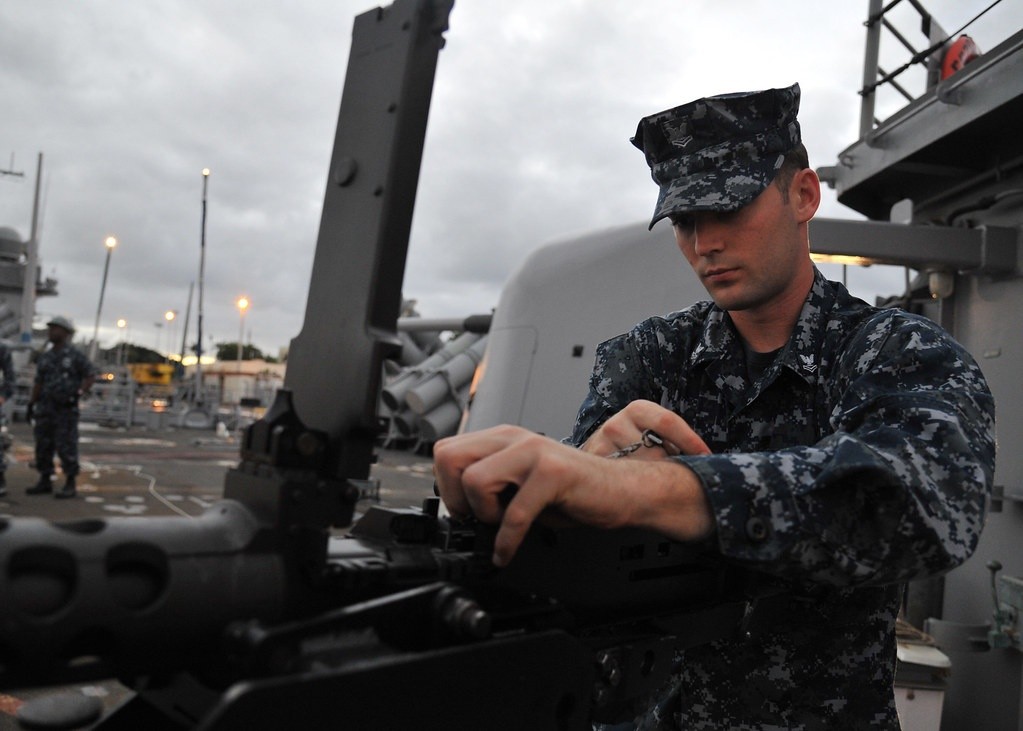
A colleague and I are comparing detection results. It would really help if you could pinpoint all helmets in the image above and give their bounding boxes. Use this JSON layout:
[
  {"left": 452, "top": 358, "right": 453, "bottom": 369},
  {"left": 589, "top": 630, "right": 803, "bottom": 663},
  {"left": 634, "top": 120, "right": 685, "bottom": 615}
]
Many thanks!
[{"left": 48, "top": 316, "right": 73, "bottom": 333}]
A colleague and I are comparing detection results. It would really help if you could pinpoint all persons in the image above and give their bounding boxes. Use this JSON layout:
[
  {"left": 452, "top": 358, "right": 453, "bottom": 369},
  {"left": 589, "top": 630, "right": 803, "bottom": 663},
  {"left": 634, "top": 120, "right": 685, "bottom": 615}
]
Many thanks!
[
  {"left": 24, "top": 314, "right": 93, "bottom": 497},
  {"left": 0, "top": 341, "right": 15, "bottom": 499},
  {"left": 432, "top": 79, "right": 995, "bottom": 731}
]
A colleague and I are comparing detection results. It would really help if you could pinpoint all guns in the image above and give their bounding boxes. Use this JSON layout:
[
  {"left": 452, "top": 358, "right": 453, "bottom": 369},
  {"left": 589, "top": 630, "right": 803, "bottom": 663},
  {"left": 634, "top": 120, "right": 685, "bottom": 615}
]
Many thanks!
[{"left": 0, "top": 0, "right": 641, "bottom": 730}]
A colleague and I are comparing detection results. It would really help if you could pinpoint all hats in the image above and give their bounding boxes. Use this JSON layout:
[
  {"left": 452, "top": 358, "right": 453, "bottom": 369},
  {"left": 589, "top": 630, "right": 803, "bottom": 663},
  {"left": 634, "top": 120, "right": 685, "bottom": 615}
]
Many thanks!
[{"left": 630, "top": 82, "right": 803, "bottom": 231}]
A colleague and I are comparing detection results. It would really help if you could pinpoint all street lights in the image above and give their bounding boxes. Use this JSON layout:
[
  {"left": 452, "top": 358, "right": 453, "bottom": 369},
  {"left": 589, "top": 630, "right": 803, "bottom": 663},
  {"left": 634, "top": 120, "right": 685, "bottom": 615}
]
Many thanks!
[
  {"left": 164, "top": 311, "right": 175, "bottom": 367},
  {"left": 114, "top": 319, "right": 125, "bottom": 367},
  {"left": 194, "top": 168, "right": 210, "bottom": 405},
  {"left": 85, "top": 236, "right": 117, "bottom": 363},
  {"left": 234, "top": 297, "right": 250, "bottom": 410}
]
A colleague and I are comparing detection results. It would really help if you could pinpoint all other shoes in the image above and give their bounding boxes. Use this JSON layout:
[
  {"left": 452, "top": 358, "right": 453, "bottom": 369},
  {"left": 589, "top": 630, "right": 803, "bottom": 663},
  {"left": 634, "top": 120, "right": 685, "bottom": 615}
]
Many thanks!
[
  {"left": 25, "top": 477, "right": 52, "bottom": 494},
  {"left": 52, "top": 480, "right": 75, "bottom": 499}
]
